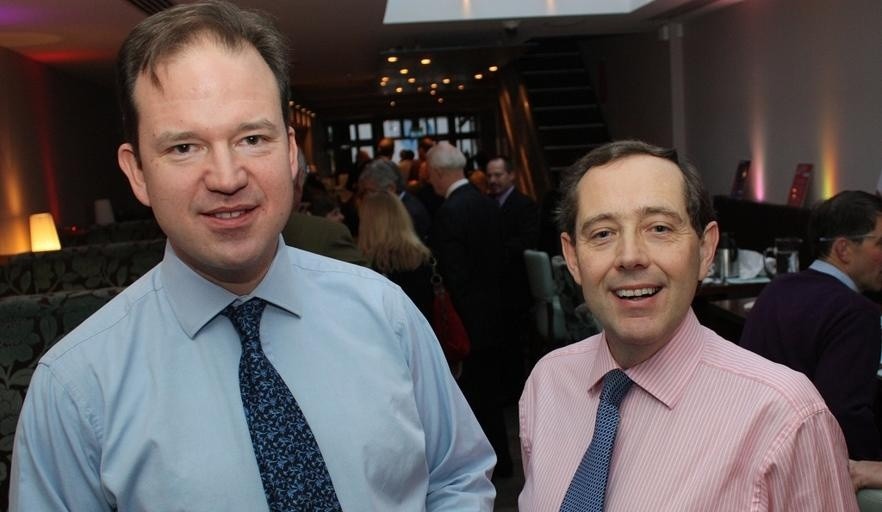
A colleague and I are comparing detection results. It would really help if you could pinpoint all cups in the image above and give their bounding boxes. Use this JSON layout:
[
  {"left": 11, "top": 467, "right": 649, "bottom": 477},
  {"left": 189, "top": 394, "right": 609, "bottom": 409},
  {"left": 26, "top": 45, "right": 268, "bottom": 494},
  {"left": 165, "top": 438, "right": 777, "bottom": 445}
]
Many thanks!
[{"left": 762, "top": 238, "right": 804, "bottom": 280}]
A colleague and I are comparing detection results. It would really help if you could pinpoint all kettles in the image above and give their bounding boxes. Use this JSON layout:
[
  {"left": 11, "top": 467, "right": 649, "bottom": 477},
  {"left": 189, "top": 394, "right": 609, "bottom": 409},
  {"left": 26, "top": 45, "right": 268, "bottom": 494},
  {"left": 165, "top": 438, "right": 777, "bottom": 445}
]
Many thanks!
[{"left": 713, "top": 232, "right": 740, "bottom": 279}]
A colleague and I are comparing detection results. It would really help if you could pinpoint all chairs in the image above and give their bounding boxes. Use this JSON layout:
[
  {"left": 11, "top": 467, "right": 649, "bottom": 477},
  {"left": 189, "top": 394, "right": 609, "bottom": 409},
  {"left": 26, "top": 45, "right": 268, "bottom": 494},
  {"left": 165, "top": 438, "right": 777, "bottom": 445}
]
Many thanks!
[{"left": 523, "top": 249, "right": 596, "bottom": 345}]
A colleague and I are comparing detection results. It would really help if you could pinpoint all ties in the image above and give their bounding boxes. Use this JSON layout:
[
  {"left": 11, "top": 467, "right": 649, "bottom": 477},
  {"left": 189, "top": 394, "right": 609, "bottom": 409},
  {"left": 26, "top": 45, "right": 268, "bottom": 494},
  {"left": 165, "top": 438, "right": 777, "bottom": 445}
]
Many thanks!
[
  {"left": 560, "top": 369, "right": 633, "bottom": 512},
  {"left": 220, "top": 300, "right": 343, "bottom": 512}
]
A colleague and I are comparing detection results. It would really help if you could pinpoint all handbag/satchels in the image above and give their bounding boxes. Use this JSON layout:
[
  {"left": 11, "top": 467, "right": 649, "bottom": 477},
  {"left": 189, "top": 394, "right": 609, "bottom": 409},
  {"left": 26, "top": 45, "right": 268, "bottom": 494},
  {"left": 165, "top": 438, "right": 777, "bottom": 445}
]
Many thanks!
[{"left": 434, "top": 289, "right": 471, "bottom": 357}]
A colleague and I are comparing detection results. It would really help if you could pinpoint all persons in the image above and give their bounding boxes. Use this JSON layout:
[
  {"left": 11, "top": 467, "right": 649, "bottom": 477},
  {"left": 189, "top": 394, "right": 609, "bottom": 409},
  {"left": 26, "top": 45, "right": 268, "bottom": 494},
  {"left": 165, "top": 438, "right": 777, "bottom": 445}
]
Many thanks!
[
  {"left": 846, "top": 456, "right": 881, "bottom": 498},
  {"left": 518, "top": 136, "right": 860, "bottom": 511},
  {"left": 5, "top": 1, "right": 499, "bottom": 511},
  {"left": 739, "top": 186, "right": 882, "bottom": 462},
  {"left": 284, "top": 130, "right": 563, "bottom": 481}
]
